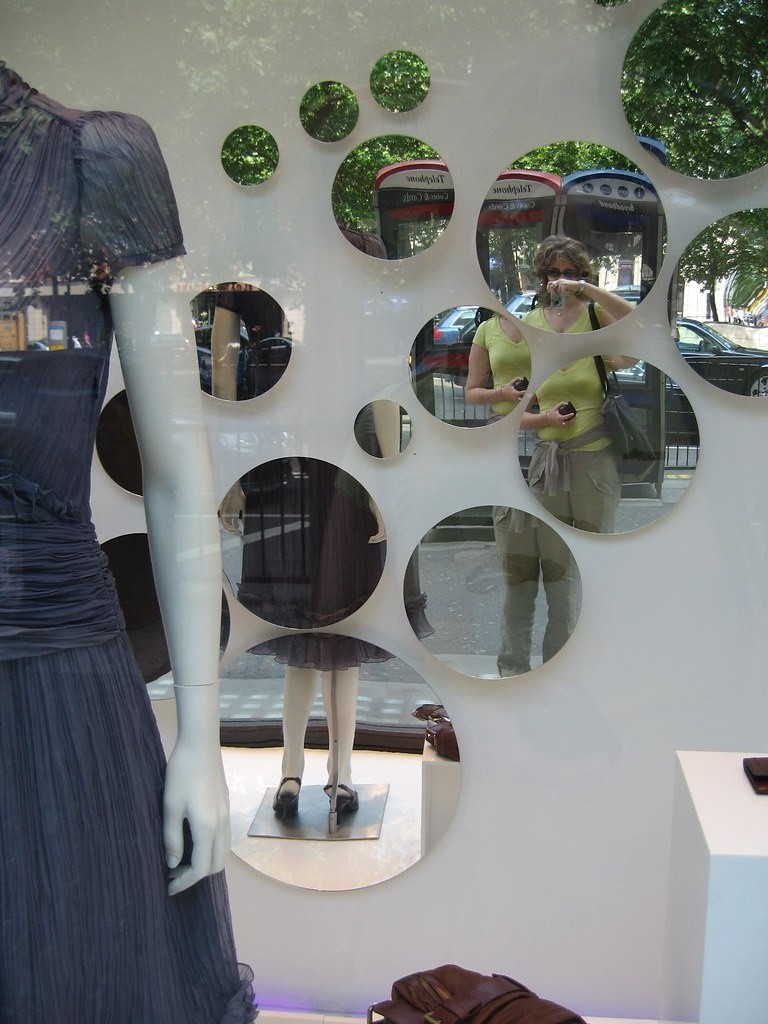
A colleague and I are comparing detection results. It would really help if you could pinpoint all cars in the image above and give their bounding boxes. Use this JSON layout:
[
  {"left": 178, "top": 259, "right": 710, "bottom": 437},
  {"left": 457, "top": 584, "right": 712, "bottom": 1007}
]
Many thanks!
[
  {"left": 610, "top": 317, "right": 767, "bottom": 446},
  {"left": 449, "top": 286, "right": 641, "bottom": 388},
  {"left": 195, "top": 326, "right": 253, "bottom": 391},
  {"left": 424, "top": 306, "right": 479, "bottom": 371}
]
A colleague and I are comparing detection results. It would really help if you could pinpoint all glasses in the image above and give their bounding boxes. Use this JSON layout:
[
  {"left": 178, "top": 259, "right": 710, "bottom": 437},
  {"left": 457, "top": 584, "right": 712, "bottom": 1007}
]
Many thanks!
[{"left": 545, "top": 266, "right": 580, "bottom": 279}]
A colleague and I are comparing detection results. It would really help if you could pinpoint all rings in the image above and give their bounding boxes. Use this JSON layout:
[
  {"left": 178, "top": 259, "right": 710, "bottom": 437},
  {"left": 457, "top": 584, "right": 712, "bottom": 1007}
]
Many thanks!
[
  {"left": 562, "top": 421, "right": 565, "bottom": 425},
  {"left": 552, "top": 281, "right": 558, "bottom": 286},
  {"left": 518, "top": 397, "right": 521, "bottom": 401}
]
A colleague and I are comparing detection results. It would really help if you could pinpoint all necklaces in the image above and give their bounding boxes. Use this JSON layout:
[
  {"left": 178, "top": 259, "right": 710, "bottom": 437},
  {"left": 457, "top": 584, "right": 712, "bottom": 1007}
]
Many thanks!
[{"left": 550, "top": 300, "right": 579, "bottom": 316}]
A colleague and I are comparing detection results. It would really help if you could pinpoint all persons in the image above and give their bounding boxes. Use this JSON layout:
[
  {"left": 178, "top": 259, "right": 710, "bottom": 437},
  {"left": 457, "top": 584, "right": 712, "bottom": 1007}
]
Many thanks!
[
  {"left": 83, "top": 331, "right": 92, "bottom": 349},
  {"left": 272, "top": 637, "right": 359, "bottom": 825},
  {"left": 220, "top": 457, "right": 386, "bottom": 628},
  {"left": 0, "top": 61, "right": 230, "bottom": 1024},
  {"left": 355, "top": 400, "right": 400, "bottom": 458},
  {"left": 464, "top": 237, "right": 641, "bottom": 678},
  {"left": 338, "top": 225, "right": 387, "bottom": 260},
  {"left": 68, "top": 336, "right": 74, "bottom": 349},
  {"left": 211, "top": 282, "right": 293, "bottom": 401}
]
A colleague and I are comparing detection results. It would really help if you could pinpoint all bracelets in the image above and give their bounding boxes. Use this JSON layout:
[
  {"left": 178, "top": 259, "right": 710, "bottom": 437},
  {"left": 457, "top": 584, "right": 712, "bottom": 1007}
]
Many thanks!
[
  {"left": 500, "top": 385, "right": 504, "bottom": 400},
  {"left": 545, "top": 410, "right": 549, "bottom": 425},
  {"left": 576, "top": 278, "right": 586, "bottom": 295}
]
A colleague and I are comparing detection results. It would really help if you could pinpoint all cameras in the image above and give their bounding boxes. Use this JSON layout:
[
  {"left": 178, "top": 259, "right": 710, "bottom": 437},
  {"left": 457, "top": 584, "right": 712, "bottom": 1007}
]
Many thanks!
[{"left": 551, "top": 293, "right": 565, "bottom": 310}]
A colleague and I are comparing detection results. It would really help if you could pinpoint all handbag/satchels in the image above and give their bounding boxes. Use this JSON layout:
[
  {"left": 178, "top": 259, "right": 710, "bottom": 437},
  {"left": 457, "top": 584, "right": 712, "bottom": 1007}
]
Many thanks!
[{"left": 600, "top": 388, "right": 654, "bottom": 485}]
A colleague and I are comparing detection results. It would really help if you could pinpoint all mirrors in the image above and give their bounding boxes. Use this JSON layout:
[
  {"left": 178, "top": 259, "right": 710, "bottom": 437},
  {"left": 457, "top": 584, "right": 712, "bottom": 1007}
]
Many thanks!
[{"left": 95, "top": 0, "right": 766, "bottom": 893}]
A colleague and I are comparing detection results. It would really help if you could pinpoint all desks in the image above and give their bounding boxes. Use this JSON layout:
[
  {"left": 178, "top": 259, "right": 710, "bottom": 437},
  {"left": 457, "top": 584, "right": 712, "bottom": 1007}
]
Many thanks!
[{"left": 664, "top": 751, "right": 768, "bottom": 1024}]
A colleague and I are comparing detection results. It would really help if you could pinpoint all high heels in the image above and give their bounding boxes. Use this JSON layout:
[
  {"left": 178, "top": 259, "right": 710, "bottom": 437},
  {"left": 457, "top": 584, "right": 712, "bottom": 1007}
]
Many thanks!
[
  {"left": 323, "top": 785, "right": 360, "bottom": 825},
  {"left": 272, "top": 777, "right": 302, "bottom": 821}
]
